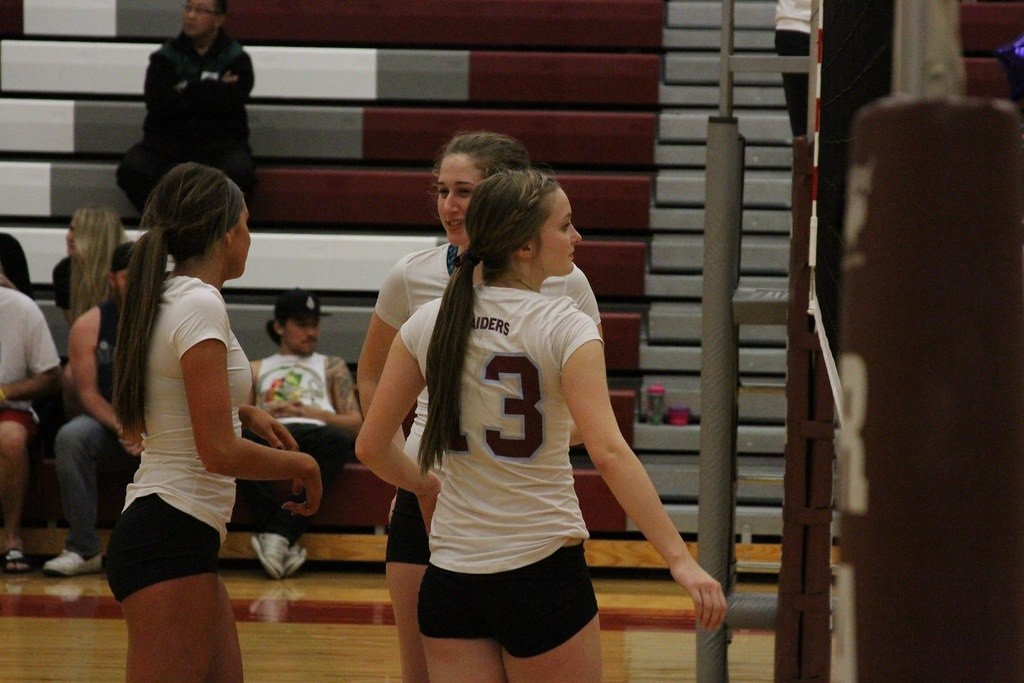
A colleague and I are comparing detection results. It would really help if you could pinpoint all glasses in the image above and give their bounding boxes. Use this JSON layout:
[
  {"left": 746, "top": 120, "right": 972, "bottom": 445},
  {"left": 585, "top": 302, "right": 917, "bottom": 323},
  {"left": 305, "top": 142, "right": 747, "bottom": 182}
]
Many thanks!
[{"left": 182, "top": 4, "right": 218, "bottom": 15}]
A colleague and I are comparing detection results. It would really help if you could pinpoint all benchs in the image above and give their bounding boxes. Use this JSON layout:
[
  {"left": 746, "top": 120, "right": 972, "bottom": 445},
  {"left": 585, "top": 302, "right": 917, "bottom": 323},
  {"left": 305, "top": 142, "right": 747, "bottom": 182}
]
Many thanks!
[{"left": 0, "top": 0, "right": 1024, "bottom": 576}]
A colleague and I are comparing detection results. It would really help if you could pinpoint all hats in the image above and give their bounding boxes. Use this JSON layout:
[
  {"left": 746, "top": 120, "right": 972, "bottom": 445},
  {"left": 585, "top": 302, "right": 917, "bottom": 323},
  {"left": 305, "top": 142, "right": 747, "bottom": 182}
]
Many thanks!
[
  {"left": 110, "top": 242, "right": 137, "bottom": 271},
  {"left": 279, "top": 290, "right": 332, "bottom": 318}
]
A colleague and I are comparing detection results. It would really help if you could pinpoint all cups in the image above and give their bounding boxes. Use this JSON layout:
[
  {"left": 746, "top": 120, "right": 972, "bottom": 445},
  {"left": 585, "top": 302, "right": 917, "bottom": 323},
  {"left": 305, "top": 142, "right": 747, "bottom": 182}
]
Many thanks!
[
  {"left": 647, "top": 384, "right": 665, "bottom": 424},
  {"left": 669, "top": 406, "right": 690, "bottom": 426}
]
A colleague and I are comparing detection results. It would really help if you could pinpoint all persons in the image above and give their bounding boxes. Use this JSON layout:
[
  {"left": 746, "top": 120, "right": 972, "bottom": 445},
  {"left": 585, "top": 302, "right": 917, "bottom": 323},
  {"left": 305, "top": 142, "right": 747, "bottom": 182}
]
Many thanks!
[
  {"left": 102, "top": 162, "right": 324, "bottom": 683},
  {"left": 355, "top": 169, "right": 728, "bottom": 683},
  {"left": 776, "top": 0, "right": 811, "bottom": 139},
  {"left": 116, "top": 0, "right": 260, "bottom": 213},
  {"left": 0, "top": 208, "right": 360, "bottom": 581},
  {"left": 359, "top": 132, "right": 601, "bottom": 683}
]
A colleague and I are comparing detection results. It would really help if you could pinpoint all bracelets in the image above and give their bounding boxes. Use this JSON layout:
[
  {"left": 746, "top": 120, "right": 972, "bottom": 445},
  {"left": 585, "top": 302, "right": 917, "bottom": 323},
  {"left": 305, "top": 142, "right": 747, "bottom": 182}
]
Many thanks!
[{"left": 0, "top": 385, "right": 5, "bottom": 400}]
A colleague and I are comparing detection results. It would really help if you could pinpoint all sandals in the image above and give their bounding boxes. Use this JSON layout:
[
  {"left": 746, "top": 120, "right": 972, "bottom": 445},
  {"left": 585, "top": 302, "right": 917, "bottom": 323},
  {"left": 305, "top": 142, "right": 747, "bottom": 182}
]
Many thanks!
[{"left": 4, "top": 549, "right": 35, "bottom": 573}]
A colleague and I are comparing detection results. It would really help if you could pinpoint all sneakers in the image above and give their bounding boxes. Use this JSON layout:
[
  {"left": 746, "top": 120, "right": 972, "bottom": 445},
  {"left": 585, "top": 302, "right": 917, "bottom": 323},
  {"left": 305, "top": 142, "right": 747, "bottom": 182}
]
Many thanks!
[
  {"left": 251, "top": 531, "right": 288, "bottom": 581},
  {"left": 286, "top": 543, "right": 308, "bottom": 576},
  {"left": 43, "top": 550, "right": 104, "bottom": 577}
]
